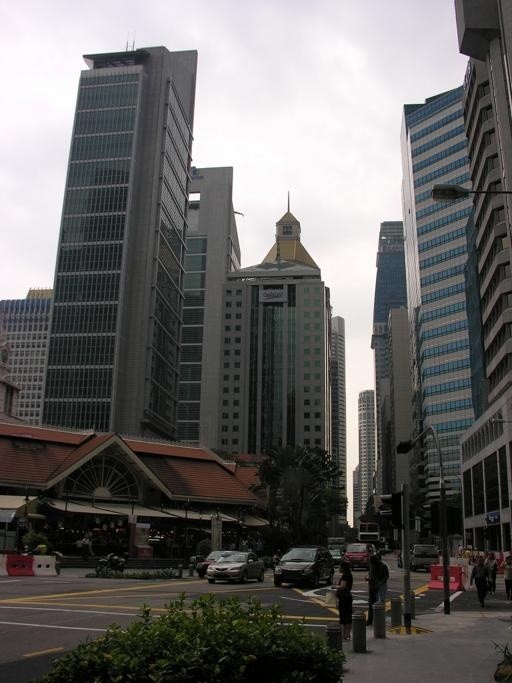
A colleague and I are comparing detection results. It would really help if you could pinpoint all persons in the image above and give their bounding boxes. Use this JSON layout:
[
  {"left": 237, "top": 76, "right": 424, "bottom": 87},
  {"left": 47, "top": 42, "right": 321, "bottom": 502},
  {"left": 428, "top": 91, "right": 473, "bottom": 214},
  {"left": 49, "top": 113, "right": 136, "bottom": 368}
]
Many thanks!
[
  {"left": 80, "top": 536, "right": 90, "bottom": 560},
  {"left": 456, "top": 544, "right": 511, "bottom": 609},
  {"left": 373, "top": 554, "right": 389, "bottom": 604},
  {"left": 363, "top": 555, "right": 384, "bottom": 626},
  {"left": 331, "top": 560, "right": 353, "bottom": 641}
]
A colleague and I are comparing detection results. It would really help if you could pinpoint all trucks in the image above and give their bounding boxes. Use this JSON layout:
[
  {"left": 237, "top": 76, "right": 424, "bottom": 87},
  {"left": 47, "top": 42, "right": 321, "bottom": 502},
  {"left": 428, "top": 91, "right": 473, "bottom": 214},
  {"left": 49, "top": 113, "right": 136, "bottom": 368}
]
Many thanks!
[
  {"left": 356, "top": 522, "right": 380, "bottom": 541},
  {"left": 327, "top": 536, "right": 353, "bottom": 551}
]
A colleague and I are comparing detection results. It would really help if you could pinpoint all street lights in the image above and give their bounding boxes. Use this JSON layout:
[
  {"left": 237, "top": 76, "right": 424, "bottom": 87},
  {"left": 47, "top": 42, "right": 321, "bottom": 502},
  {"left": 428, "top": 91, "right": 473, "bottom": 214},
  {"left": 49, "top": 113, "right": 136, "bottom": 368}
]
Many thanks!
[
  {"left": 428, "top": 180, "right": 512, "bottom": 203},
  {"left": 396, "top": 425, "right": 450, "bottom": 615},
  {"left": 490, "top": 416, "right": 511, "bottom": 425}
]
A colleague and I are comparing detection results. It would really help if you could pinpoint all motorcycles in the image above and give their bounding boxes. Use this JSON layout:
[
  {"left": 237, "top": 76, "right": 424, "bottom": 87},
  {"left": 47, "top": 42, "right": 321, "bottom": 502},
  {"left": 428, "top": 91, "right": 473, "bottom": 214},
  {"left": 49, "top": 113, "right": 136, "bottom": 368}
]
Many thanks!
[
  {"left": 96, "top": 553, "right": 125, "bottom": 575},
  {"left": 21, "top": 548, "right": 63, "bottom": 573},
  {"left": 271, "top": 553, "right": 279, "bottom": 568}
]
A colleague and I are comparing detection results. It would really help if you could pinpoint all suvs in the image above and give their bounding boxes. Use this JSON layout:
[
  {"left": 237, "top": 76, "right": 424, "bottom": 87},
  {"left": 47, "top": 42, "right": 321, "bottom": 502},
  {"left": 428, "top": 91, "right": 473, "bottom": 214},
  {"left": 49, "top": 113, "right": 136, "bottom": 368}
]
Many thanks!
[
  {"left": 343, "top": 543, "right": 379, "bottom": 571},
  {"left": 397, "top": 543, "right": 439, "bottom": 572},
  {"left": 273, "top": 548, "right": 334, "bottom": 586},
  {"left": 328, "top": 549, "right": 343, "bottom": 565}
]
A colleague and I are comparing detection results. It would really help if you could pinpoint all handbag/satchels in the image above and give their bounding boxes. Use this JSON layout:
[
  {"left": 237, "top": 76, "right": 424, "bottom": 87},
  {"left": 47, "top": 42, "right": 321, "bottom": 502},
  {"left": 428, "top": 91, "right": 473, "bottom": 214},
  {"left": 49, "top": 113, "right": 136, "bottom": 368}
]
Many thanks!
[{"left": 325, "top": 590, "right": 338, "bottom": 608}]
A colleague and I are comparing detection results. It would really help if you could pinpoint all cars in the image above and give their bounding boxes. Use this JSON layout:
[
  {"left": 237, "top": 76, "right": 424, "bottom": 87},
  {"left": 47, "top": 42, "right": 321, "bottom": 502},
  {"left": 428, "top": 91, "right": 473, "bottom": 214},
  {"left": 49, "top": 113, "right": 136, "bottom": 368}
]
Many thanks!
[
  {"left": 207, "top": 552, "right": 265, "bottom": 583},
  {"left": 369, "top": 541, "right": 393, "bottom": 560},
  {"left": 197, "top": 550, "right": 225, "bottom": 576}
]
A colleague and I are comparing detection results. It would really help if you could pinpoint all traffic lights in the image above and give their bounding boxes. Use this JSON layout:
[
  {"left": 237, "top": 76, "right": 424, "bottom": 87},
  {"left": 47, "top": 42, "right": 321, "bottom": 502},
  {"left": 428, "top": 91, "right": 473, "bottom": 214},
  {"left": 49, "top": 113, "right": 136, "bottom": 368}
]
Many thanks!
[{"left": 378, "top": 491, "right": 398, "bottom": 528}]
